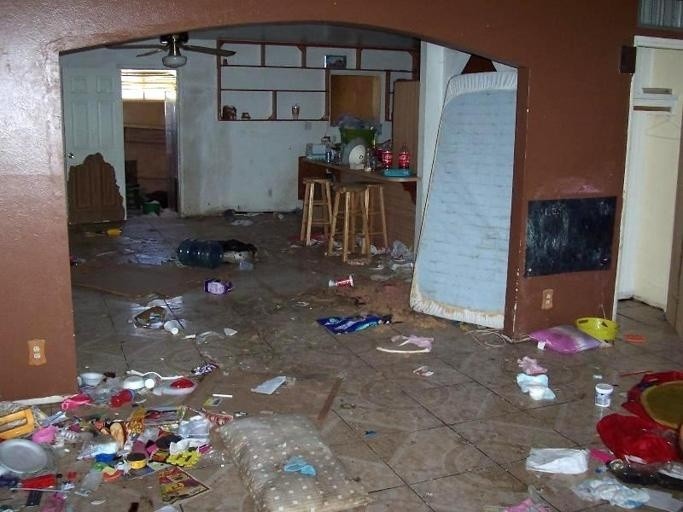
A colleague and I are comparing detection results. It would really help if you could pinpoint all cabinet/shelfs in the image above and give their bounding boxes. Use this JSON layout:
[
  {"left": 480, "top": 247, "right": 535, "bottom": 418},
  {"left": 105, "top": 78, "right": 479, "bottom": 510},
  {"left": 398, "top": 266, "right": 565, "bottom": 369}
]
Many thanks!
[{"left": 208, "top": 38, "right": 421, "bottom": 129}]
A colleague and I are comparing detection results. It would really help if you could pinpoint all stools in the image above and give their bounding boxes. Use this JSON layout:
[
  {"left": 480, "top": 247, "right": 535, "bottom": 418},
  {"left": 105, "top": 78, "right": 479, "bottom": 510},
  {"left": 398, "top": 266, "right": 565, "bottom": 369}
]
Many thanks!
[{"left": 295, "top": 170, "right": 391, "bottom": 266}]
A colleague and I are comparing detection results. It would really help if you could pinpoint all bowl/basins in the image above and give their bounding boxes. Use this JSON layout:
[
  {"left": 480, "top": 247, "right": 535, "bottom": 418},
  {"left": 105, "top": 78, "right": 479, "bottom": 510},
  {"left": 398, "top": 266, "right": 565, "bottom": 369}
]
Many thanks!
[
  {"left": 121, "top": 376, "right": 143, "bottom": 390},
  {"left": 80, "top": 372, "right": 105, "bottom": 386},
  {"left": 576, "top": 317, "right": 617, "bottom": 341},
  {"left": 143, "top": 202, "right": 160, "bottom": 215},
  {"left": 340, "top": 126, "right": 376, "bottom": 143}
]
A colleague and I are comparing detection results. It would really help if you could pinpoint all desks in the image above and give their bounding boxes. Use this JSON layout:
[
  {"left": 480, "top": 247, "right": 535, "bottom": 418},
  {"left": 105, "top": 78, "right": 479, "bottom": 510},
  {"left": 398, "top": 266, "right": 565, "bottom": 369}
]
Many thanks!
[{"left": 292, "top": 149, "right": 418, "bottom": 254}]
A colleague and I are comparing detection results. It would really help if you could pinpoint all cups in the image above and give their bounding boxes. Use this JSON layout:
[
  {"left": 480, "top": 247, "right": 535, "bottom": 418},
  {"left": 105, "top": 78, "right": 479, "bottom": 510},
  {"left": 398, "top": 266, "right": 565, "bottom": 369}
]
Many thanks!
[
  {"left": 163, "top": 320, "right": 179, "bottom": 335},
  {"left": 145, "top": 376, "right": 155, "bottom": 389},
  {"left": 363, "top": 148, "right": 392, "bottom": 172},
  {"left": 328, "top": 275, "right": 354, "bottom": 288},
  {"left": 291, "top": 106, "right": 299, "bottom": 116},
  {"left": 325, "top": 152, "right": 331, "bottom": 163}
]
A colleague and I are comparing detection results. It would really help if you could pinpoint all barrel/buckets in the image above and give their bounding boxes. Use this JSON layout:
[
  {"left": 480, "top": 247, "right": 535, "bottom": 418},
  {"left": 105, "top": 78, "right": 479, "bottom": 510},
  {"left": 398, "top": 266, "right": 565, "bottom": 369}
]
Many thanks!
[
  {"left": 176, "top": 238, "right": 224, "bottom": 270},
  {"left": 338, "top": 126, "right": 377, "bottom": 146}
]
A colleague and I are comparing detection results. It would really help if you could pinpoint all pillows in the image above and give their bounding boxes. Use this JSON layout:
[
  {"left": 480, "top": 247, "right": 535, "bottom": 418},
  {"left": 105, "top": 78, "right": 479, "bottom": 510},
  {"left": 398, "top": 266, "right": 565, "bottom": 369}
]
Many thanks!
[{"left": 205, "top": 408, "right": 377, "bottom": 511}]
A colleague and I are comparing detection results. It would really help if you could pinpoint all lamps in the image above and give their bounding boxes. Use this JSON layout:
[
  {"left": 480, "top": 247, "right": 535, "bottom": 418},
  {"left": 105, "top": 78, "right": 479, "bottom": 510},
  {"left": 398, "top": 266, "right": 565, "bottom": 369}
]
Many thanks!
[{"left": 158, "top": 52, "right": 190, "bottom": 70}]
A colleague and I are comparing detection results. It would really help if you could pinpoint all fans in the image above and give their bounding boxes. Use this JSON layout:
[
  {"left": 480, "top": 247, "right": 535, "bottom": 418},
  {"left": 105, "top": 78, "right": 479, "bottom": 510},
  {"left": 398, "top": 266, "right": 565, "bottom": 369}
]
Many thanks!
[{"left": 99, "top": 31, "right": 239, "bottom": 62}]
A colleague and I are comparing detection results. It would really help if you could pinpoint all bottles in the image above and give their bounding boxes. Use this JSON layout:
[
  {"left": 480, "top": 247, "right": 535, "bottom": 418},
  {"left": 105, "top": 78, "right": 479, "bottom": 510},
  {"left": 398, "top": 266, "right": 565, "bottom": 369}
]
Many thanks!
[
  {"left": 97, "top": 229, "right": 123, "bottom": 236},
  {"left": 71, "top": 255, "right": 79, "bottom": 281},
  {"left": 398, "top": 144, "right": 409, "bottom": 169}
]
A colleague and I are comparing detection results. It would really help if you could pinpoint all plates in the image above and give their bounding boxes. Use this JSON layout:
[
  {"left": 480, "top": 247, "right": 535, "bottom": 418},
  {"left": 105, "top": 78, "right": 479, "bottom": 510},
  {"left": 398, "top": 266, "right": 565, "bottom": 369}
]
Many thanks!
[{"left": 0, "top": 439, "right": 47, "bottom": 473}]
